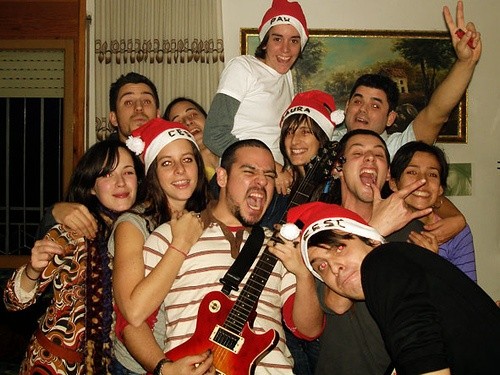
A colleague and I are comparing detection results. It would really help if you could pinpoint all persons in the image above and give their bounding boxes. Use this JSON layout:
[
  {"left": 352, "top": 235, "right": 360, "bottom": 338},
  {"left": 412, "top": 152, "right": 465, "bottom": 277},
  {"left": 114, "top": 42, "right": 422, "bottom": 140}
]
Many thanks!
[
  {"left": 0, "top": 134, "right": 145, "bottom": 375},
  {"left": 115, "top": 138, "right": 326, "bottom": 375},
  {"left": 108, "top": 0, "right": 484, "bottom": 285},
  {"left": 281, "top": 201, "right": 500, "bottom": 375},
  {"left": 314, "top": 128, "right": 439, "bottom": 375}
]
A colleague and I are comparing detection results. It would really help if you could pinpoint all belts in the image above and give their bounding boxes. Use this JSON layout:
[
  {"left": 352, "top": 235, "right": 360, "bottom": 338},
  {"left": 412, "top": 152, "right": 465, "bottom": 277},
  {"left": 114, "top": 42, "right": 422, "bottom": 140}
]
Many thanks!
[{"left": 36, "top": 330, "right": 83, "bottom": 363}]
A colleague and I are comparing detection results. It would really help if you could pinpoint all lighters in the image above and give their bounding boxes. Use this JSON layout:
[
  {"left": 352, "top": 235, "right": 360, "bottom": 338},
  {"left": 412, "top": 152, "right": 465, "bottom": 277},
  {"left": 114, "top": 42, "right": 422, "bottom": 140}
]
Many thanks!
[{"left": 455, "top": 28, "right": 476, "bottom": 50}]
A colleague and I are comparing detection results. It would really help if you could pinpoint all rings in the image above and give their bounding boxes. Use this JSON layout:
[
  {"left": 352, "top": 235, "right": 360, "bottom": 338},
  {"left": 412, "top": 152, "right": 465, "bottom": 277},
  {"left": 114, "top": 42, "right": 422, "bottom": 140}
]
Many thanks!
[
  {"left": 196, "top": 213, "right": 201, "bottom": 218},
  {"left": 293, "top": 241, "right": 300, "bottom": 248},
  {"left": 286, "top": 188, "right": 291, "bottom": 192}
]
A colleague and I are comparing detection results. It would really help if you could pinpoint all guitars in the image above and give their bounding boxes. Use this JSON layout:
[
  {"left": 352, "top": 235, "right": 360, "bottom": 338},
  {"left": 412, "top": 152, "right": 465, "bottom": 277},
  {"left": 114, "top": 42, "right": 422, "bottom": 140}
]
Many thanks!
[{"left": 144, "top": 140, "right": 347, "bottom": 375}]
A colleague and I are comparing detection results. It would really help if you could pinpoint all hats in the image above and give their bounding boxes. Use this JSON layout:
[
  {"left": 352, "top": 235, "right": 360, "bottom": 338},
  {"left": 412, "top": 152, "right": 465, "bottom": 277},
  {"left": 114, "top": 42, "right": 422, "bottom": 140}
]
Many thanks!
[
  {"left": 280, "top": 90, "right": 345, "bottom": 140},
  {"left": 258, "top": 0, "right": 309, "bottom": 52},
  {"left": 126, "top": 118, "right": 200, "bottom": 176},
  {"left": 280, "top": 202, "right": 386, "bottom": 282}
]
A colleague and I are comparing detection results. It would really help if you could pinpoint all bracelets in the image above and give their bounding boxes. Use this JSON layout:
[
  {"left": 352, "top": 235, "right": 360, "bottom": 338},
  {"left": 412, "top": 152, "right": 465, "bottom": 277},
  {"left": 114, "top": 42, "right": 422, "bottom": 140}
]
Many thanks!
[
  {"left": 25, "top": 260, "right": 41, "bottom": 280},
  {"left": 169, "top": 245, "right": 187, "bottom": 257}
]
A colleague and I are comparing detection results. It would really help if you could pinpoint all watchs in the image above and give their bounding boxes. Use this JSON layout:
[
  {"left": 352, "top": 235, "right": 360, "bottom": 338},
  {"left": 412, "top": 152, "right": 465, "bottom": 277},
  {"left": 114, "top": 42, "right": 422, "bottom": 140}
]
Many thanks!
[{"left": 153, "top": 357, "right": 174, "bottom": 375}]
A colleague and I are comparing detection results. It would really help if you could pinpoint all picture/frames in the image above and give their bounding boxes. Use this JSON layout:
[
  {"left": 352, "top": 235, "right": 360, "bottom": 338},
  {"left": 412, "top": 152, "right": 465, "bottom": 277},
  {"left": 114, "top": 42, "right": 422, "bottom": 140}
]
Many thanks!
[{"left": 241, "top": 28, "right": 468, "bottom": 145}]
